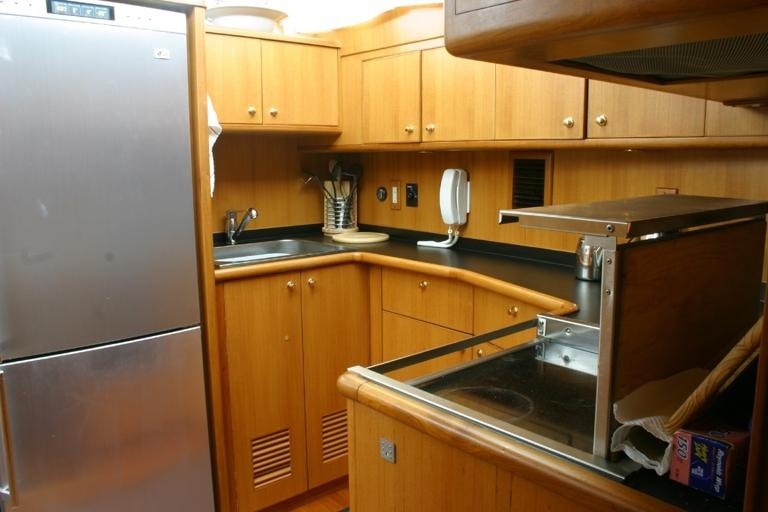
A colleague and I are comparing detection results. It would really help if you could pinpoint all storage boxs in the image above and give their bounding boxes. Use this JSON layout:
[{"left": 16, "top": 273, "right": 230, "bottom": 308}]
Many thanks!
[{"left": 666, "top": 425, "right": 751, "bottom": 505}]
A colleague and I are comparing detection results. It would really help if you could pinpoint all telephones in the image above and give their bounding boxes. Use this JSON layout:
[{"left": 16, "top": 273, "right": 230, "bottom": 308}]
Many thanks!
[{"left": 439, "top": 168, "right": 468, "bottom": 226}]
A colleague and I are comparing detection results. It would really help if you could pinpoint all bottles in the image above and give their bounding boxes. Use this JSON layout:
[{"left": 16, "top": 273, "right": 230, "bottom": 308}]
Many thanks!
[{"left": 575, "top": 237, "right": 605, "bottom": 281}]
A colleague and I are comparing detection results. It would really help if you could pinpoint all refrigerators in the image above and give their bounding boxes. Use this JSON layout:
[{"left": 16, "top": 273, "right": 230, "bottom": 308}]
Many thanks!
[{"left": 0, "top": 0, "right": 219, "bottom": 512}]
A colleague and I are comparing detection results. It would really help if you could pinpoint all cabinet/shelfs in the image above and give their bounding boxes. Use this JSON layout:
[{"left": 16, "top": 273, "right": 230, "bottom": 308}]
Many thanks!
[
  {"left": 216, "top": 262, "right": 368, "bottom": 497},
  {"left": 212, "top": 237, "right": 768, "bottom": 512},
  {"left": 199, "top": 1, "right": 768, "bottom": 151},
  {"left": 364, "top": 254, "right": 566, "bottom": 385}
]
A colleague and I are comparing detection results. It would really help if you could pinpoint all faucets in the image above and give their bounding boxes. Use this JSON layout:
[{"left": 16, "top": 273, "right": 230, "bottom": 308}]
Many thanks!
[{"left": 225, "top": 206, "right": 257, "bottom": 244}]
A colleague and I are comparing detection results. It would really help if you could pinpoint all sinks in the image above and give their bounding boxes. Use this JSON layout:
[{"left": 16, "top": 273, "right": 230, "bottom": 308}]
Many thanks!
[{"left": 213, "top": 239, "right": 354, "bottom": 268}]
